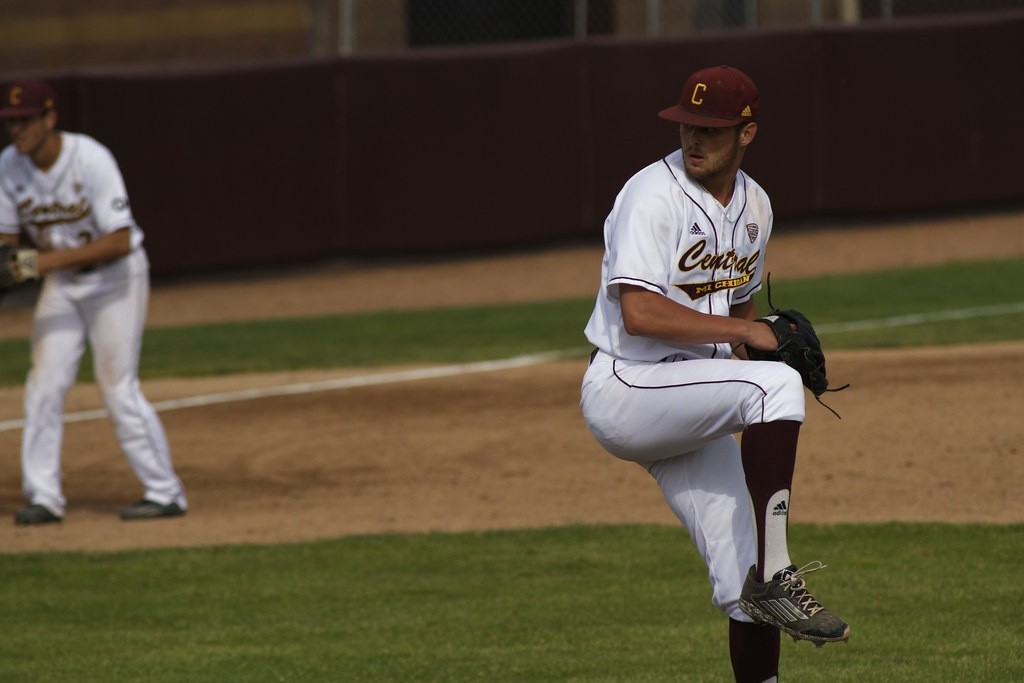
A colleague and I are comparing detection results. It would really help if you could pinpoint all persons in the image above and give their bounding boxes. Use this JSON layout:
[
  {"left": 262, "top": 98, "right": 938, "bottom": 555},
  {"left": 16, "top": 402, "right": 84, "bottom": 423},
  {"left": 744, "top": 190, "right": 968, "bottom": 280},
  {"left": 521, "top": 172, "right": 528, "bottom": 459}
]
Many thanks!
[
  {"left": 0, "top": 78, "right": 188, "bottom": 525},
  {"left": 580, "top": 65, "right": 850, "bottom": 683}
]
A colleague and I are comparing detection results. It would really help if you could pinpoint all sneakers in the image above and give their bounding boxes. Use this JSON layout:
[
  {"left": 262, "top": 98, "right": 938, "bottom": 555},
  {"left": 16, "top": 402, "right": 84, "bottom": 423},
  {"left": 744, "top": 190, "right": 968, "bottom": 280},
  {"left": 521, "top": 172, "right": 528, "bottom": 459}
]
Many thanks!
[{"left": 738, "top": 563, "right": 851, "bottom": 647}]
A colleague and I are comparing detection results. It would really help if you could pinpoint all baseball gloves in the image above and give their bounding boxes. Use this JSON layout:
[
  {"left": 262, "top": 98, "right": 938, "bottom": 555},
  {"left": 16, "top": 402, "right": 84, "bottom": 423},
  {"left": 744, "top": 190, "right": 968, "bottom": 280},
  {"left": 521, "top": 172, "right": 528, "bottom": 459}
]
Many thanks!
[
  {"left": 0, "top": 241, "right": 42, "bottom": 299},
  {"left": 743, "top": 308, "right": 830, "bottom": 400}
]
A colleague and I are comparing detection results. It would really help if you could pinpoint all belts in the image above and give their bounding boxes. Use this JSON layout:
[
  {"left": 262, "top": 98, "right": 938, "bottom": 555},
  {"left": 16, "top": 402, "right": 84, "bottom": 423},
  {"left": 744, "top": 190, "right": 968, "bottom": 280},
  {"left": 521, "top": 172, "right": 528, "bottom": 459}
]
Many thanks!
[
  {"left": 77, "top": 261, "right": 111, "bottom": 272},
  {"left": 589, "top": 347, "right": 688, "bottom": 364}
]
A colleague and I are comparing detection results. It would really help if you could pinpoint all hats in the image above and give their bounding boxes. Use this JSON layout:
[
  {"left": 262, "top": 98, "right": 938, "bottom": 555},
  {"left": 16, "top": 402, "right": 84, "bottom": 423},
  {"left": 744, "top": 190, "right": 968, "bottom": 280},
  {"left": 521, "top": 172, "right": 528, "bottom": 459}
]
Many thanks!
[
  {"left": 658, "top": 66, "right": 760, "bottom": 128},
  {"left": 1, "top": 78, "right": 55, "bottom": 118}
]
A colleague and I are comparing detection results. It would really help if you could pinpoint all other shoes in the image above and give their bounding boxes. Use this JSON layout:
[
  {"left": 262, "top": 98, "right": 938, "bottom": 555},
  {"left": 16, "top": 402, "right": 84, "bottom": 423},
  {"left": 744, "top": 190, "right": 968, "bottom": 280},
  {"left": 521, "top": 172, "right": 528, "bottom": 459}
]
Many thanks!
[
  {"left": 123, "top": 501, "right": 183, "bottom": 518},
  {"left": 16, "top": 506, "right": 53, "bottom": 522}
]
